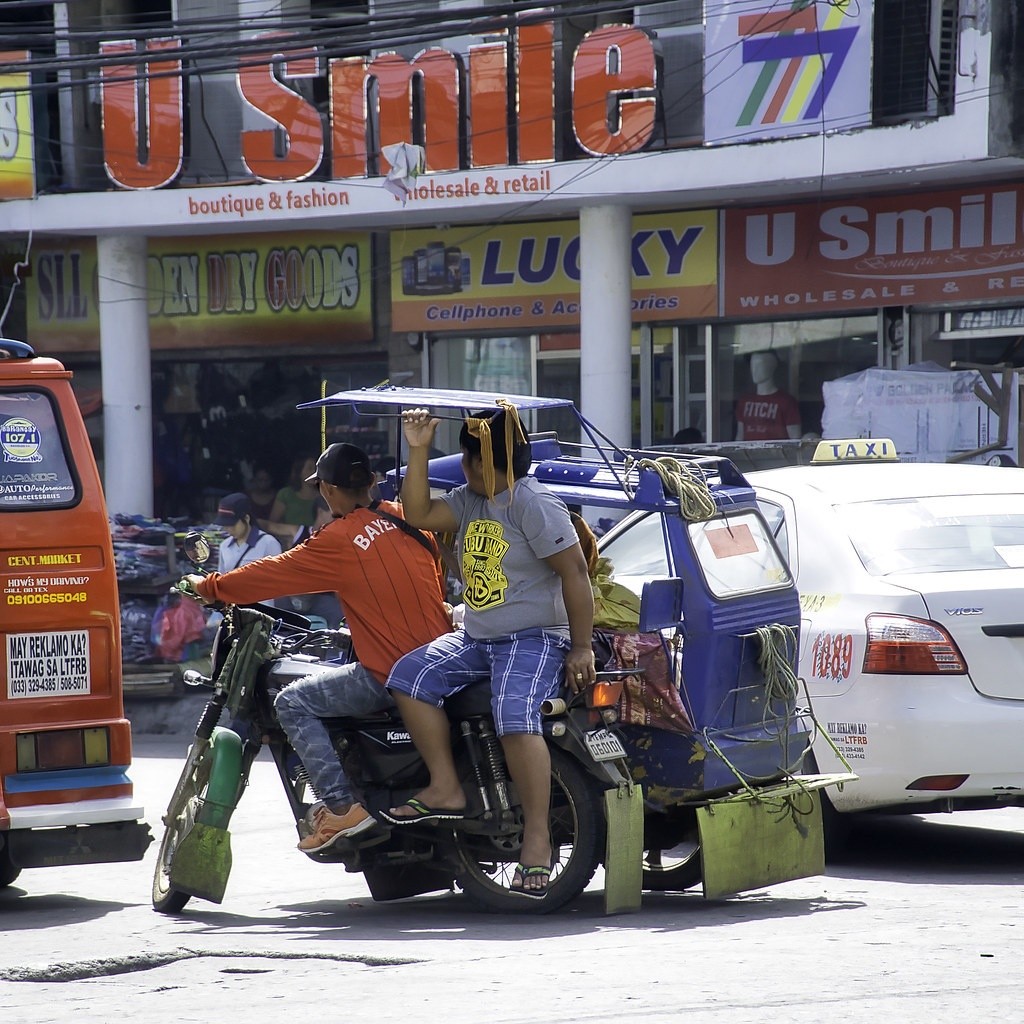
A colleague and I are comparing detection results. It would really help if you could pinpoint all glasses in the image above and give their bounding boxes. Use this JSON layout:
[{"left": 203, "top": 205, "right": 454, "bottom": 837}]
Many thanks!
[{"left": 314, "top": 478, "right": 338, "bottom": 490}]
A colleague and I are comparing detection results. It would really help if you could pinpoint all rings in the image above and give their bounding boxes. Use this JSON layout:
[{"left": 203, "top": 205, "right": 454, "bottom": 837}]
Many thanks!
[{"left": 574, "top": 673, "right": 582, "bottom": 679}]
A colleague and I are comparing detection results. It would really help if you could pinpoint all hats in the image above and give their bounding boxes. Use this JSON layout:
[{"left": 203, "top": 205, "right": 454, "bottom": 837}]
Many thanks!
[
  {"left": 304, "top": 443, "right": 375, "bottom": 492},
  {"left": 212, "top": 492, "right": 255, "bottom": 525}
]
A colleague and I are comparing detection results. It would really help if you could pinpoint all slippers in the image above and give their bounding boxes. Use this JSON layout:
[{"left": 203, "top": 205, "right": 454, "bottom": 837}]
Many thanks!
[
  {"left": 509, "top": 847, "right": 554, "bottom": 900},
  {"left": 378, "top": 796, "right": 464, "bottom": 824}
]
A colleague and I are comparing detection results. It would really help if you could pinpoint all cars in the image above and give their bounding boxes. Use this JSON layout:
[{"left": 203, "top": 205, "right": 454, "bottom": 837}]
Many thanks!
[
  {"left": 0, "top": 332, "right": 157, "bottom": 887},
  {"left": 733, "top": 442, "right": 1024, "bottom": 833}
]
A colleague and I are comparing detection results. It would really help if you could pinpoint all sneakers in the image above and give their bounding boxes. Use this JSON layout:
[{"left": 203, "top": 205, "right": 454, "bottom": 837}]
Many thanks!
[{"left": 297, "top": 802, "right": 377, "bottom": 853}]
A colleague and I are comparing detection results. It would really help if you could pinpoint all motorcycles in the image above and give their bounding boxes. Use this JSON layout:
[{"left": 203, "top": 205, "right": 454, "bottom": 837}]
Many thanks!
[{"left": 144, "top": 386, "right": 860, "bottom": 919}]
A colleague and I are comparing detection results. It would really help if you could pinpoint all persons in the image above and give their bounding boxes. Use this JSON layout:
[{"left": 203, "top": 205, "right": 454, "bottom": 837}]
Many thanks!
[
  {"left": 732, "top": 342, "right": 802, "bottom": 442},
  {"left": 377, "top": 408, "right": 595, "bottom": 899},
  {"left": 211, "top": 493, "right": 281, "bottom": 607},
  {"left": 262, "top": 454, "right": 323, "bottom": 612},
  {"left": 239, "top": 456, "right": 280, "bottom": 520},
  {"left": 184, "top": 535, "right": 202, "bottom": 560},
  {"left": 312, "top": 495, "right": 334, "bottom": 533},
  {"left": 180, "top": 442, "right": 455, "bottom": 853}
]
